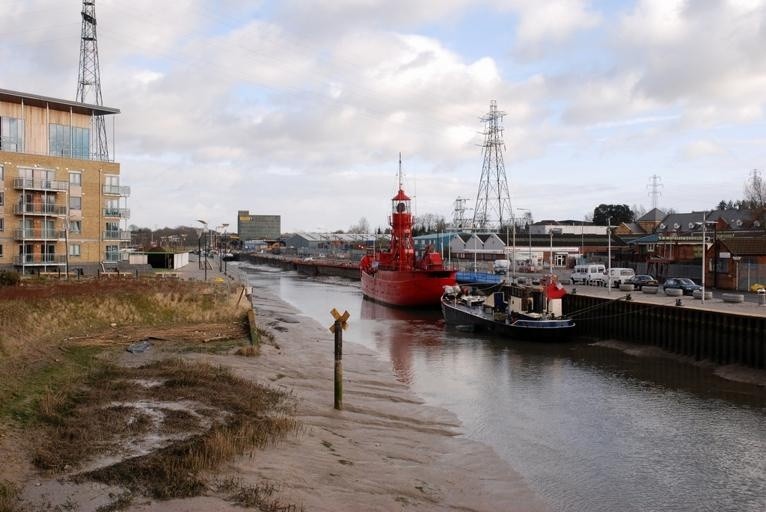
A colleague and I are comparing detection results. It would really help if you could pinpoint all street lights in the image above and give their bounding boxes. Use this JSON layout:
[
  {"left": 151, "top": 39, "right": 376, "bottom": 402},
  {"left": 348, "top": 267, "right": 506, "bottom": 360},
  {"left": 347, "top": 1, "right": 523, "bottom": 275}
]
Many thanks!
[
  {"left": 694, "top": 212, "right": 718, "bottom": 304},
  {"left": 604, "top": 215, "right": 615, "bottom": 292},
  {"left": 547, "top": 229, "right": 554, "bottom": 275},
  {"left": 195, "top": 219, "right": 230, "bottom": 280},
  {"left": 515, "top": 207, "right": 532, "bottom": 266}
]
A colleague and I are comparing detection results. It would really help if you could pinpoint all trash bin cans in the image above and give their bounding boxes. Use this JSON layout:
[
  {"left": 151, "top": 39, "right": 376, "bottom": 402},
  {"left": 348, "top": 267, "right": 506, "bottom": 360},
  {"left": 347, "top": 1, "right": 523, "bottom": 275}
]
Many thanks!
[{"left": 757, "top": 288, "right": 766, "bottom": 304}]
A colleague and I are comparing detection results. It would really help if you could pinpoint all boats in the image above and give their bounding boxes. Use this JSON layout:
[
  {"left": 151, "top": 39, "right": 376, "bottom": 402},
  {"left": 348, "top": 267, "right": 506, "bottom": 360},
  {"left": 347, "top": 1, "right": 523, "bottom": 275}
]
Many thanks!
[
  {"left": 197, "top": 247, "right": 234, "bottom": 262},
  {"left": 436, "top": 281, "right": 577, "bottom": 342}
]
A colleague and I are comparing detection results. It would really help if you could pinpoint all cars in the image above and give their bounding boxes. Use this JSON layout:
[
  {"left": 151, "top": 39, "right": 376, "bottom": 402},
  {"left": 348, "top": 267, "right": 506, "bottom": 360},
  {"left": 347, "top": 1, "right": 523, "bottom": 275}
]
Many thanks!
[
  {"left": 662, "top": 277, "right": 704, "bottom": 297},
  {"left": 491, "top": 259, "right": 512, "bottom": 274},
  {"left": 623, "top": 275, "right": 660, "bottom": 293}
]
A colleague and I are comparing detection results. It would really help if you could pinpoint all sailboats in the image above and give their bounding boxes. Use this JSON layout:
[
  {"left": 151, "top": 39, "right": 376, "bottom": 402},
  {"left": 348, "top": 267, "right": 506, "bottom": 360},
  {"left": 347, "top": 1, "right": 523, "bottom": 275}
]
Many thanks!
[{"left": 359, "top": 151, "right": 458, "bottom": 308}]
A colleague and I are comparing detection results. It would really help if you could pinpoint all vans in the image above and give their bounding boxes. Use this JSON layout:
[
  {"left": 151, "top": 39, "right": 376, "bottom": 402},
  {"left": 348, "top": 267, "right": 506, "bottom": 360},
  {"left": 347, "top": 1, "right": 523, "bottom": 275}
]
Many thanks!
[
  {"left": 569, "top": 264, "right": 606, "bottom": 286},
  {"left": 601, "top": 267, "right": 635, "bottom": 289}
]
